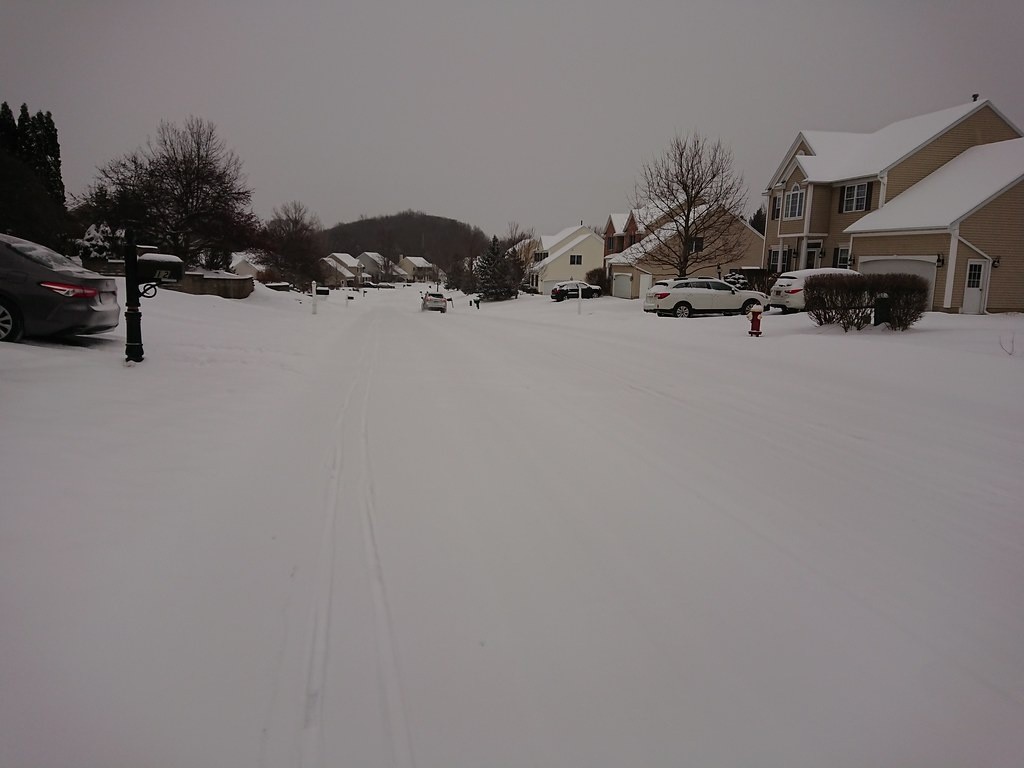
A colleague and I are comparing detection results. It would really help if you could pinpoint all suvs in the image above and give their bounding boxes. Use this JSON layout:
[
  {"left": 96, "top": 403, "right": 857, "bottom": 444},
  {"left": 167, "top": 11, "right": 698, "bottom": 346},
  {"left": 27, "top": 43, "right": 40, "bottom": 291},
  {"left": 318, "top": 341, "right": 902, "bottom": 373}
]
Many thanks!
[
  {"left": 643, "top": 274, "right": 771, "bottom": 319},
  {"left": 550, "top": 279, "right": 603, "bottom": 302},
  {"left": 420, "top": 292, "right": 447, "bottom": 313},
  {"left": 768, "top": 266, "right": 889, "bottom": 315}
]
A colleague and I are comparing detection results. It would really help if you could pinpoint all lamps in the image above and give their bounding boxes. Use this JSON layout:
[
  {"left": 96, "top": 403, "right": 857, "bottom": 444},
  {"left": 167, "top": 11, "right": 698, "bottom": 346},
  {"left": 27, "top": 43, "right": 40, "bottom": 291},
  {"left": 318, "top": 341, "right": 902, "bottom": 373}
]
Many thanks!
[
  {"left": 847, "top": 254, "right": 855, "bottom": 266},
  {"left": 992, "top": 259, "right": 1000, "bottom": 268},
  {"left": 819, "top": 248, "right": 826, "bottom": 258},
  {"left": 792, "top": 249, "right": 797, "bottom": 258},
  {"left": 936, "top": 254, "right": 944, "bottom": 268}
]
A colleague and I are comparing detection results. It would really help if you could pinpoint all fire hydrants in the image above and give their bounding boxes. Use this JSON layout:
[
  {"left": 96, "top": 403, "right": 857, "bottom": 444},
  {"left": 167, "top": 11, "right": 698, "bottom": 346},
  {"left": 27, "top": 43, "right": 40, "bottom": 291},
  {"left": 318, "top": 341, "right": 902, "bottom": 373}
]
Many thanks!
[{"left": 747, "top": 305, "right": 764, "bottom": 337}]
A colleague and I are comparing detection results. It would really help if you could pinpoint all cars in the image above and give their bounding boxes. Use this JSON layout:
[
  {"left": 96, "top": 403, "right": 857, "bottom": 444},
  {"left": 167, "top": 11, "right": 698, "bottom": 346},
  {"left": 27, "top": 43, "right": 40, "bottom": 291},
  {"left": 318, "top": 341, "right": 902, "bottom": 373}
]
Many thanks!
[
  {"left": 359, "top": 281, "right": 395, "bottom": 289},
  {"left": 0, "top": 231, "right": 123, "bottom": 346}
]
